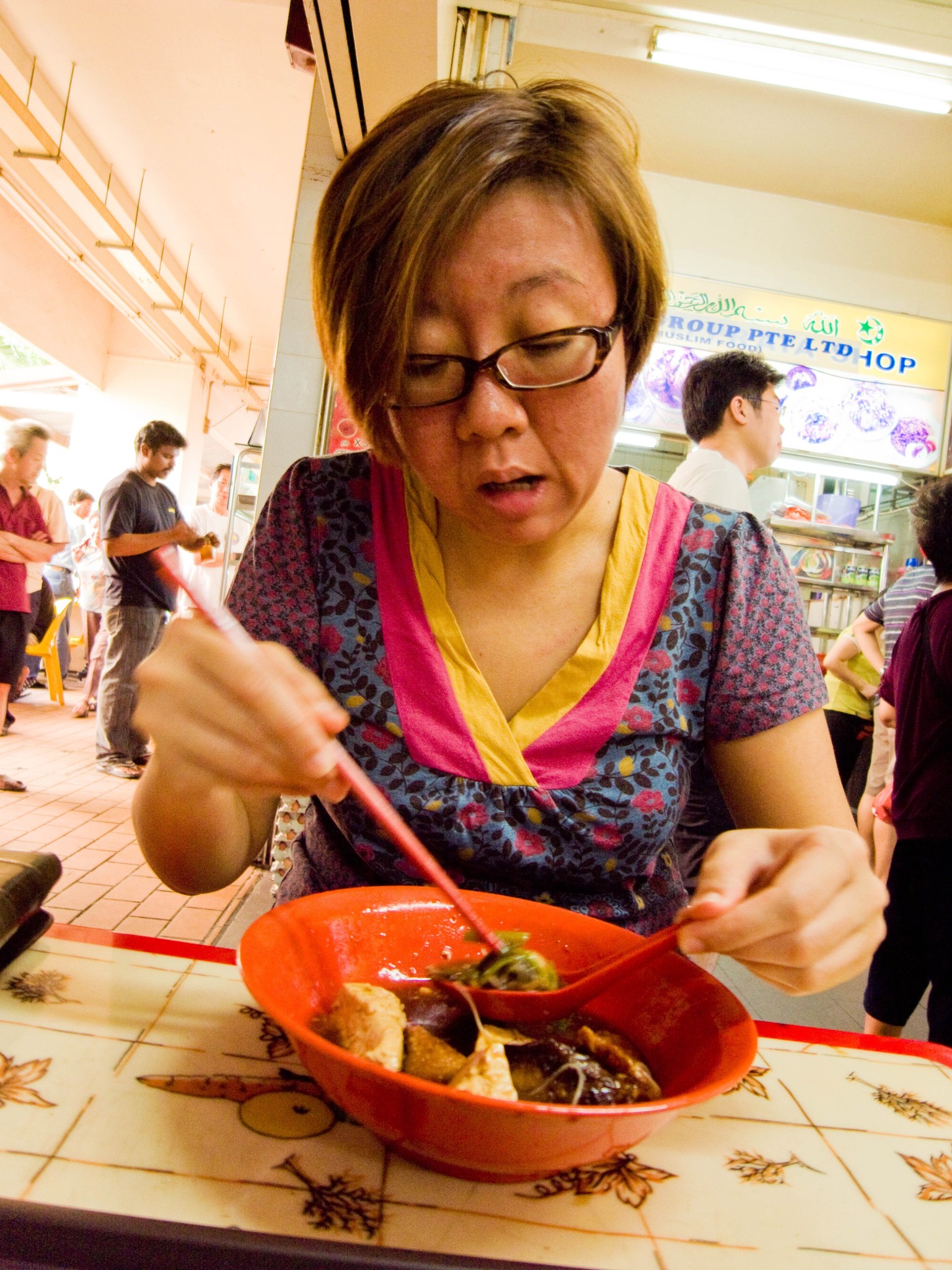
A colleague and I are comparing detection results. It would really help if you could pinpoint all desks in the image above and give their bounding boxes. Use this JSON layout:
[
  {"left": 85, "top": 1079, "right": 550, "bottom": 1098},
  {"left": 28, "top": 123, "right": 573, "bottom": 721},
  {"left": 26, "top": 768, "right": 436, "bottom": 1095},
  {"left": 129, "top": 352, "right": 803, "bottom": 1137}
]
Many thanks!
[{"left": 0, "top": 922, "right": 952, "bottom": 1270}]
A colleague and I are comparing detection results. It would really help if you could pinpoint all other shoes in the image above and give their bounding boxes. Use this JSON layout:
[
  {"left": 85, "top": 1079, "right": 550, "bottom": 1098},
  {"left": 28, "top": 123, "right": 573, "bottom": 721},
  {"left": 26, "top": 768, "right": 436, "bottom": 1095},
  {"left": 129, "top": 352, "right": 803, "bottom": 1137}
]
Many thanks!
[
  {"left": 0, "top": 772, "right": 25, "bottom": 791},
  {"left": 72, "top": 703, "right": 88, "bottom": 719}
]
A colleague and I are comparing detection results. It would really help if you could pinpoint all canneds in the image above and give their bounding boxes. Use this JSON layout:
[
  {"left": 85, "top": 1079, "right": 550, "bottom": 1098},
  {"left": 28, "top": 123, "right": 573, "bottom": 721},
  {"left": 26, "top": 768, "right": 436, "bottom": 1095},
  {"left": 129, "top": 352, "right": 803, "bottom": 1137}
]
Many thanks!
[{"left": 841, "top": 565, "right": 879, "bottom": 588}]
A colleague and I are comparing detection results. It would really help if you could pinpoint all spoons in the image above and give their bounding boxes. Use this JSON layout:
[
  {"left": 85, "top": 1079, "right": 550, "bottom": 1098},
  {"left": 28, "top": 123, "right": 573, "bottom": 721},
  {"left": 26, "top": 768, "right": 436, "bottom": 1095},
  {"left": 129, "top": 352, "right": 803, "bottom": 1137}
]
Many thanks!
[{"left": 432, "top": 917, "right": 694, "bottom": 1020}]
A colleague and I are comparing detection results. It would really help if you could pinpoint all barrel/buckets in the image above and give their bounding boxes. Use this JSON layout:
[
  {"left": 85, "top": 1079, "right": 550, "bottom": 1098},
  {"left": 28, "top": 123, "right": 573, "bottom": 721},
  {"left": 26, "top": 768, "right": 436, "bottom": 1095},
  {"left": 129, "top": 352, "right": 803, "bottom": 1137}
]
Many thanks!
[{"left": 816, "top": 493, "right": 860, "bottom": 529}]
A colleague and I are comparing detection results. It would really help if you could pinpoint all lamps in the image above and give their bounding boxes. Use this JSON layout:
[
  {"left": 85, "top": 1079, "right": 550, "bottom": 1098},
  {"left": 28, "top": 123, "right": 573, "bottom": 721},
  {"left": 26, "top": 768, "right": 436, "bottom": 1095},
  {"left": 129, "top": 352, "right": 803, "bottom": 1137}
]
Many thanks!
[{"left": 646, "top": 22, "right": 952, "bottom": 115}]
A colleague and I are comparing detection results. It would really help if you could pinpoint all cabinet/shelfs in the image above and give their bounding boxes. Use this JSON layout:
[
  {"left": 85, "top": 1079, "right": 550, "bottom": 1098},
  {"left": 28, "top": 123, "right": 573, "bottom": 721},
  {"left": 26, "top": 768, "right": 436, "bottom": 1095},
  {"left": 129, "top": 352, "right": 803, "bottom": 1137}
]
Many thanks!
[{"left": 769, "top": 515, "right": 896, "bottom": 679}]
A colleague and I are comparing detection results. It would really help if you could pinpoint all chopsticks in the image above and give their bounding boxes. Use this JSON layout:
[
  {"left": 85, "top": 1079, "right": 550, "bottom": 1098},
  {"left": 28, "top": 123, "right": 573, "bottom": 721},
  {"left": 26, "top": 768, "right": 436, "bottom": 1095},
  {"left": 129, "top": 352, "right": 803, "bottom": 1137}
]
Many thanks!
[{"left": 155, "top": 546, "right": 508, "bottom": 959}]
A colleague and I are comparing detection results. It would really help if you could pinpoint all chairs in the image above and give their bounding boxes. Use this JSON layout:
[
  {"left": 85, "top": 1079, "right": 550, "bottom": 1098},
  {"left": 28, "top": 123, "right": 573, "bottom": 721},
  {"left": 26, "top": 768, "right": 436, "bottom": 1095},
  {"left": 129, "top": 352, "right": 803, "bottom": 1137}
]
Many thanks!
[{"left": 26, "top": 596, "right": 73, "bottom": 706}]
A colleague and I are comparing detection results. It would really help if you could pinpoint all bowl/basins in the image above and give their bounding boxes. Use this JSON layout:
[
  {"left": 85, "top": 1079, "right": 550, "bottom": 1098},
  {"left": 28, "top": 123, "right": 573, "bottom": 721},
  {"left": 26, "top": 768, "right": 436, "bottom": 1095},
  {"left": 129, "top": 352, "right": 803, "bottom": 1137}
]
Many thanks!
[{"left": 238, "top": 886, "right": 758, "bottom": 1182}]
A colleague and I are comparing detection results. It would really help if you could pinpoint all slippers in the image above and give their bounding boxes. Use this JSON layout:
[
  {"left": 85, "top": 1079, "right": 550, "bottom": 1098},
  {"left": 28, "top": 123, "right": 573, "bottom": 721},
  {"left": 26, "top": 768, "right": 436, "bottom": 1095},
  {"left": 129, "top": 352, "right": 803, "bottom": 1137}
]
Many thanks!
[{"left": 98, "top": 756, "right": 142, "bottom": 778}]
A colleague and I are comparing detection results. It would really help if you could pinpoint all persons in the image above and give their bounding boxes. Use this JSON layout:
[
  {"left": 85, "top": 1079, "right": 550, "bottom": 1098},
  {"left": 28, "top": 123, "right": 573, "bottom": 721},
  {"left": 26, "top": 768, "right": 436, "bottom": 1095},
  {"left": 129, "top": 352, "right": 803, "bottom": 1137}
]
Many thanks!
[
  {"left": 666, "top": 351, "right": 784, "bottom": 512},
  {"left": 823, "top": 474, "right": 952, "bottom": 1047},
  {"left": 0, "top": 421, "right": 237, "bottom": 793},
  {"left": 132, "top": 69, "right": 889, "bottom": 995}
]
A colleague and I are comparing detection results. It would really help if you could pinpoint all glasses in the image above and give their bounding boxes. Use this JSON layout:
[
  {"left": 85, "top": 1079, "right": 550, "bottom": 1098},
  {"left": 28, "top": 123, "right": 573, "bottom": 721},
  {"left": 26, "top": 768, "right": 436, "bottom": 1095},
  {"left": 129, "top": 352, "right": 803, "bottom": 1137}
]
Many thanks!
[
  {"left": 379, "top": 312, "right": 622, "bottom": 408},
  {"left": 746, "top": 396, "right": 788, "bottom": 420}
]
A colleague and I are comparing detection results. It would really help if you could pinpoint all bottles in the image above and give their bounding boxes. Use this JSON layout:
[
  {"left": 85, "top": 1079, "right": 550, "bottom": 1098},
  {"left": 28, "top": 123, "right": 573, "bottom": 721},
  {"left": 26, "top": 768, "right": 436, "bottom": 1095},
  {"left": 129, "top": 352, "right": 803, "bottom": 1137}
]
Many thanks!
[{"left": 803, "top": 558, "right": 918, "bottom": 630}]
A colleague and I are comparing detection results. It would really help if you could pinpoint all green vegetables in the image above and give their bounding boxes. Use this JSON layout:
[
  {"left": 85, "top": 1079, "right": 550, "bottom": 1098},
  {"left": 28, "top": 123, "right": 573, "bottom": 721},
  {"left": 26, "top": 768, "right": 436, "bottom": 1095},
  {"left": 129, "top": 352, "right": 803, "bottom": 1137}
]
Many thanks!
[{"left": 426, "top": 930, "right": 564, "bottom": 990}]
